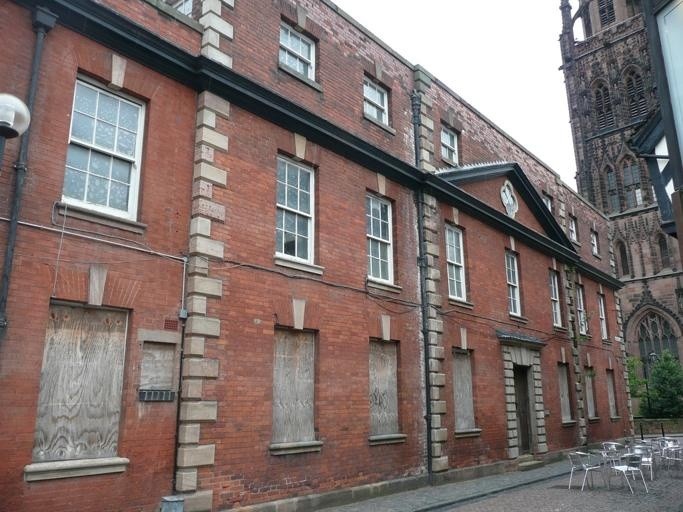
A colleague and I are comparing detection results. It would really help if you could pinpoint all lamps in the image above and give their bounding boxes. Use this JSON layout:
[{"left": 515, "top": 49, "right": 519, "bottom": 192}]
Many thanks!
[{"left": 0, "top": 90, "right": 31, "bottom": 140}]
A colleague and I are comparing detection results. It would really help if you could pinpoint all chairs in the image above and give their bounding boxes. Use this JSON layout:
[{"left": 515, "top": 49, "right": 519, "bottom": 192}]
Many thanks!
[{"left": 569, "top": 436, "right": 681, "bottom": 494}]
